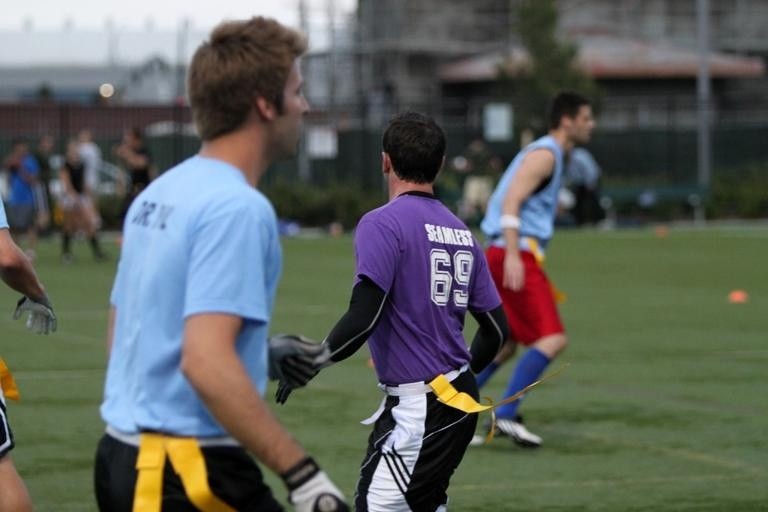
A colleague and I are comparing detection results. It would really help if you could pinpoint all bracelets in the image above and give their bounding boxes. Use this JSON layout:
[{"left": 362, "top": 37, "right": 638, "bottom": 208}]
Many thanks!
[{"left": 497, "top": 214, "right": 523, "bottom": 231}]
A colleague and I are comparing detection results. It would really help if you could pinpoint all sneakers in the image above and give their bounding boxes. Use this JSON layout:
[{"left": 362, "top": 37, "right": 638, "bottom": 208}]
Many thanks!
[{"left": 484, "top": 414, "right": 543, "bottom": 449}]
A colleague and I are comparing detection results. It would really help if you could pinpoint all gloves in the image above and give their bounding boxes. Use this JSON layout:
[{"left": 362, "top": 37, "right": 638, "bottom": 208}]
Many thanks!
[
  {"left": 269, "top": 331, "right": 332, "bottom": 405},
  {"left": 282, "top": 456, "right": 351, "bottom": 511},
  {"left": 13, "top": 295, "right": 57, "bottom": 335}
]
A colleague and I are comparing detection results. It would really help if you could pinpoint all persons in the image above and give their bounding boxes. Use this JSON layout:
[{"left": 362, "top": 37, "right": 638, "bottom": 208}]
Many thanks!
[
  {"left": 453, "top": 130, "right": 506, "bottom": 227},
  {"left": 0, "top": 195, "right": 60, "bottom": 510},
  {"left": 93, "top": 17, "right": 358, "bottom": 511},
  {"left": 463, "top": 91, "right": 598, "bottom": 453},
  {"left": 276, "top": 110, "right": 511, "bottom": 512},
  {"left": 565, "top": 143, "right": 615, "bottom": 231},
  {"left": 0, "top": 120, "right": 160, "bottom": 267}
]
